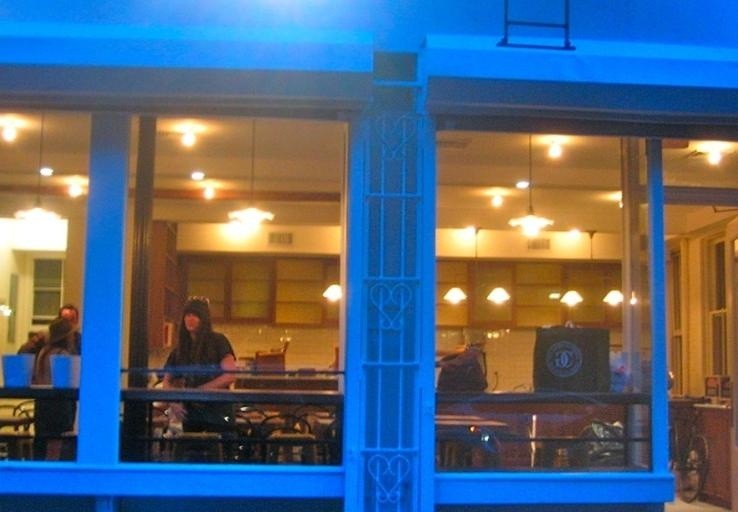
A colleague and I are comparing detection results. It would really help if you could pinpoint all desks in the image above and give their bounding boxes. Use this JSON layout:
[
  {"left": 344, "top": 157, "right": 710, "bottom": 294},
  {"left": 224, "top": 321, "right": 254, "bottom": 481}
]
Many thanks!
[{"left": 434, "top": 414, "right": 509, "bottom": 473}]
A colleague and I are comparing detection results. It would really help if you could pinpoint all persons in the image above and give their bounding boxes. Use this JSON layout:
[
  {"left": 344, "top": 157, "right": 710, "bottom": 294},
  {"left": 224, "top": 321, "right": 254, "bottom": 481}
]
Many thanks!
[
  {"left": 155, "top": 297, "right": 243, "bottom": 464},
  {"left": 31, "top": 316, "right": 78, "bottom": 460},
  {"left": 31, "top": 330, "right": 47, "bottom": 355},
  {"left": 16, "top": 331, "right": 40, "bottom": 355},
  {"left": 150, "top": 372, "right": 187, "bottom": 458},
  {"left": 432, "top": 338, "right": 490, "bottom": 397},
  {"left": 56, "top": 304, "right": 82, "bottom": 460},
  {"left": 152, "top": 368, "right": 166, "bottom": 387}
]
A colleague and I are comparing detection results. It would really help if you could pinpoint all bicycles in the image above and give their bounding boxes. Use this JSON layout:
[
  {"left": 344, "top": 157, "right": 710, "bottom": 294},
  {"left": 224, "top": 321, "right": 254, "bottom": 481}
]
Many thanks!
[{"left": 573, "top": 396, "right": 716, "bottom": 505}]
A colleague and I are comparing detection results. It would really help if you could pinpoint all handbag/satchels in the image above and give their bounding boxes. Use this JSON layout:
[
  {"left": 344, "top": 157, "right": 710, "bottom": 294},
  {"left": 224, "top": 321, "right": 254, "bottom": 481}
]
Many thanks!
[
  {"left": 435, "top": 349, "right": 489, "bottom": 393},
  {"left": 533, "top": 325, "right": 613, "bottom": 394}
]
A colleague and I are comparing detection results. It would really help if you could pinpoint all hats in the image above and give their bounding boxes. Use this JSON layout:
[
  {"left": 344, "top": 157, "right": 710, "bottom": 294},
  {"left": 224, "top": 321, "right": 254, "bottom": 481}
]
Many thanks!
[{"left": 49, "top": 316, "right": 80, "bottom": 344}]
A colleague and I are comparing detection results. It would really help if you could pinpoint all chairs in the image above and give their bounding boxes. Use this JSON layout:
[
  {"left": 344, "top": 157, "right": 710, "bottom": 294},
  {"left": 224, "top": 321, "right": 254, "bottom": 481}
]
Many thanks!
[{"left": 153, "top": 401, "right": 333, "bottom": 460}]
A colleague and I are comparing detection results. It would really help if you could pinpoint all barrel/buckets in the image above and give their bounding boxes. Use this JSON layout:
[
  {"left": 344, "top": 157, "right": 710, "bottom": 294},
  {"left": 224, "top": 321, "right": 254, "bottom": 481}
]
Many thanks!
[
  {"left": 49, "top": 352, "right": 81, "bottom": 389},
  {"left": 2, "top": 352, "right": 35, "bottom": 388}
]
